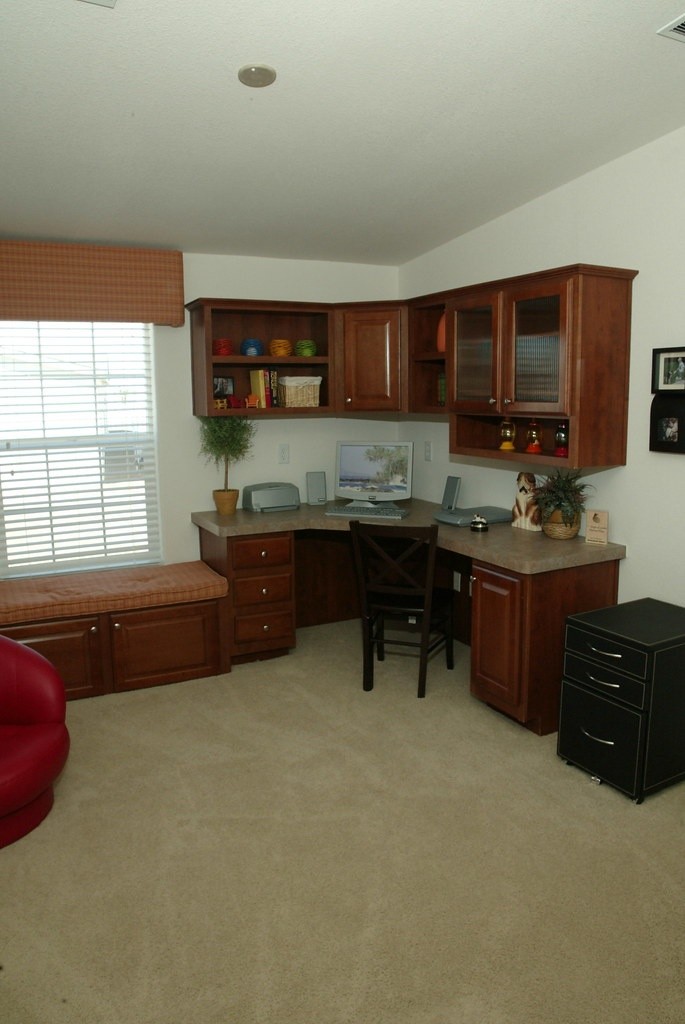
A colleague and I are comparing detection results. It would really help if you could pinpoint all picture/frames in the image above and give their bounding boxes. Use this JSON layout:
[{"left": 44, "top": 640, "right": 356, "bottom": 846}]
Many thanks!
[{"left": 650, "top": 346, "right": 685, "bottom": 394}]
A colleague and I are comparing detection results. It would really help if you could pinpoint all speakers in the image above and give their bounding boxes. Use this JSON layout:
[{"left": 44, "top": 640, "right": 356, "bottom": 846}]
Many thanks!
[
  {"left": 442, "top": 476, "right": 461, "bottom": 511},
  {"left": 306, "top": 472, "right": 327, "bottom": 505}
]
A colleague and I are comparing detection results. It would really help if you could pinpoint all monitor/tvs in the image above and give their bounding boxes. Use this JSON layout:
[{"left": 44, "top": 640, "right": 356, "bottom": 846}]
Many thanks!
[{"left": 334, "top": 440, "right": 413, "bottom": 509}]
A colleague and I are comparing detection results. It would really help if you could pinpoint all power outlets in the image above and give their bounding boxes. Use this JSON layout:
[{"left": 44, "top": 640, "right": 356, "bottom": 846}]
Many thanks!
[
  {"left": 278, "top": 444, "right": 290, "bottom": 464},
  {"left": 424, "top": 439, "right": 433, "bottom": 462}
]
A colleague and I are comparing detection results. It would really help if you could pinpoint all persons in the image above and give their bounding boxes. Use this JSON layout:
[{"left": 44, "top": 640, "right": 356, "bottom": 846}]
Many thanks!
[
  {"left": 671, "top": 357, "right": 685, "bottom": 377},
  {"left": 664, "top": 418, "right": 678, "bottom": 442}
]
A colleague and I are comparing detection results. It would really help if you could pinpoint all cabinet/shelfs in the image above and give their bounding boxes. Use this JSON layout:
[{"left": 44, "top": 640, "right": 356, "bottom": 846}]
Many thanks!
[
  {"left": 0, "top": 601, "right": 221, "bottom": 699},
  {"left": 185, "top": 297, "right": 334, "bottom": 416},
  {"left": 471, "top": 558, "right": 625, "bottom": 735},
  {"left": 327, "top": 300, "right": 411, "bottom": 413},
  {"left": 197, "top": 533, "right": 298, "bottom": 662},
  {"left": 450, "top": 264, "right": 639, "bottom": 471},
  {"left": 407, "top": 298, "right": 456, "bottom": 412},
  {"left": 557, "top": 597, "right": 685, "bottom": 804}
]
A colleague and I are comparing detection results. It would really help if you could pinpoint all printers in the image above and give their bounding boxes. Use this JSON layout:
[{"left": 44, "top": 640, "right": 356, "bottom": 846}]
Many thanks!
[{"left": 242, "top": 481, "right": 301, "bottom": 513}]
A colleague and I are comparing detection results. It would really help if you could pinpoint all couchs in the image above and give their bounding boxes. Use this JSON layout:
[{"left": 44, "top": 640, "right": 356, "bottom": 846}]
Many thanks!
[{"left": 0, "top": 634, "right": 71, "bottom": 848}]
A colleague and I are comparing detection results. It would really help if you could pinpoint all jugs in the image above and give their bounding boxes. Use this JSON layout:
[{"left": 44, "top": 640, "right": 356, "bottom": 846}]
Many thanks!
[
  {"left": 525, "top": 421, "right": 542, "bottom": 453},
  {"left": 498, "top": 420, "right": 515, "bottom": 451},
  {"left": 554, "top": 424, "right": 568, "bottom": 457}
]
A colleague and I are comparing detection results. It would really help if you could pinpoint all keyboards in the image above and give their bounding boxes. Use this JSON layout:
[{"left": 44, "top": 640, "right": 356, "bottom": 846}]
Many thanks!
[{"left": 325, "top": 506, "right": 408, "bottom": 520}]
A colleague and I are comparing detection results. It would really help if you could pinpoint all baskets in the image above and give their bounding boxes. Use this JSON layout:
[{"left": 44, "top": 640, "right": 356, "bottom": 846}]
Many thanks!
[{"left": 278, "top": 375, "right": 322, "bottom": 408}]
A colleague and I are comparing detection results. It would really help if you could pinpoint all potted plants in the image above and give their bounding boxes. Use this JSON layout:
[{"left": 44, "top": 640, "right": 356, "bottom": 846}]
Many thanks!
[
  {"left": 201, "top": 418, "right": 252, "bottom": 517},
  {"left": 533, "top": 470, "right": 595, "bottom": 541}
]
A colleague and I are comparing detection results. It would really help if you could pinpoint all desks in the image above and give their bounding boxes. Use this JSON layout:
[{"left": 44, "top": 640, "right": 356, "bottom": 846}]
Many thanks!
[{"left": 192, "top": 495, "right": 625, "bottom": 575}]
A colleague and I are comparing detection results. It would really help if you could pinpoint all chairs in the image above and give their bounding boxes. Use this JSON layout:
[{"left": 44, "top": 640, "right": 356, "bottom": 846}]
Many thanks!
[{"left": 347, "top": 520, "right": 454, "bottom": 699}]
